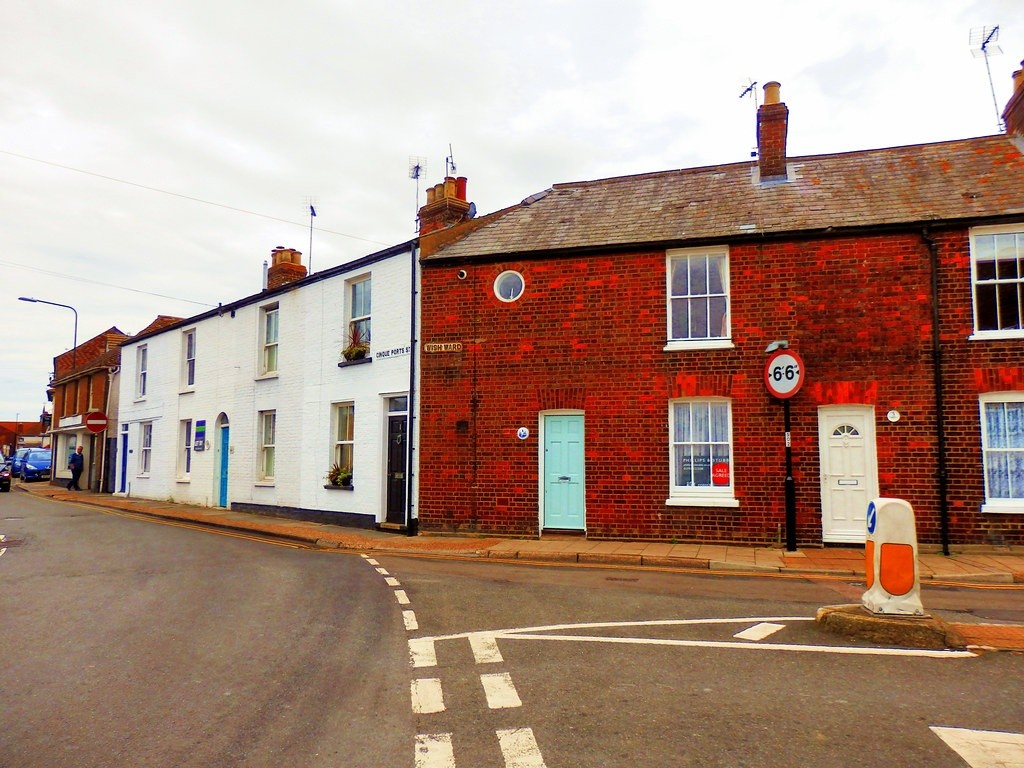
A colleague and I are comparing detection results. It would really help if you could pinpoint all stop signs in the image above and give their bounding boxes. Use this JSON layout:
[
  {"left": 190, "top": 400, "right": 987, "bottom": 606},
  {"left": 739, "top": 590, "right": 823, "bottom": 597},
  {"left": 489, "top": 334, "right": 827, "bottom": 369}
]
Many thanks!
[{"left": 84, "top": 412, "right": 108, "bottom": 433}]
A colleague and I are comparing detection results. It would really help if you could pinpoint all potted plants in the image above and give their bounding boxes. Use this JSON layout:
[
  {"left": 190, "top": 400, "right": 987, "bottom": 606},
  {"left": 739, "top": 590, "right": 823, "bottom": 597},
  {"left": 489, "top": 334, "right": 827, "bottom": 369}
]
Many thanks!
[
  {"left": 338, "top": 321, "right": 370, "bottom": 361},
  {"left": 323, "top": 462, "right": 353, "bottom": 486}
]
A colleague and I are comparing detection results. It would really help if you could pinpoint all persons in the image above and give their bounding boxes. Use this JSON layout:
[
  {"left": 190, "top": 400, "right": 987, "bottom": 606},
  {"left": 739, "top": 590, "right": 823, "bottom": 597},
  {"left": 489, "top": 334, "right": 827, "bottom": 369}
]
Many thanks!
[{"left": 66, "top": 446, "right": 84, "bottom": 491}]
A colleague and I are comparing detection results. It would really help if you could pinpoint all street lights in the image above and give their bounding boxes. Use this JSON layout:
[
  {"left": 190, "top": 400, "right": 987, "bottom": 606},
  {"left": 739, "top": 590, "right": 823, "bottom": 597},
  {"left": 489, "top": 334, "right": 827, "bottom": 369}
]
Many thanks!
[{"left": 18, "top": 297, "right": 78, "bottom": 367}]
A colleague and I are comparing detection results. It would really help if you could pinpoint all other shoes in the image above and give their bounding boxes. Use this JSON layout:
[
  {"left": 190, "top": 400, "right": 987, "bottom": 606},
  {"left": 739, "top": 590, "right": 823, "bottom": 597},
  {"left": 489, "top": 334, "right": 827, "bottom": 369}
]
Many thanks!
[{"left": 64, "top": 485, "right": 83, "bottom": 491}]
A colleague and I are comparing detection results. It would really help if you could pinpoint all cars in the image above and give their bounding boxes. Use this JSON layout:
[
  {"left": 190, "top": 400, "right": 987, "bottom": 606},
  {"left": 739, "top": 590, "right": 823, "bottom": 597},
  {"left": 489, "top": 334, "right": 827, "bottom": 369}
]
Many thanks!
[
  {"left": 0, "top": 450, "right": 11, "bottom": 492},
  {"left": 11, "top": 447, "right": 33, "bottom": 476},
  {"left": 19, "top": 448, "right": 52, "bottom": 482}
]
